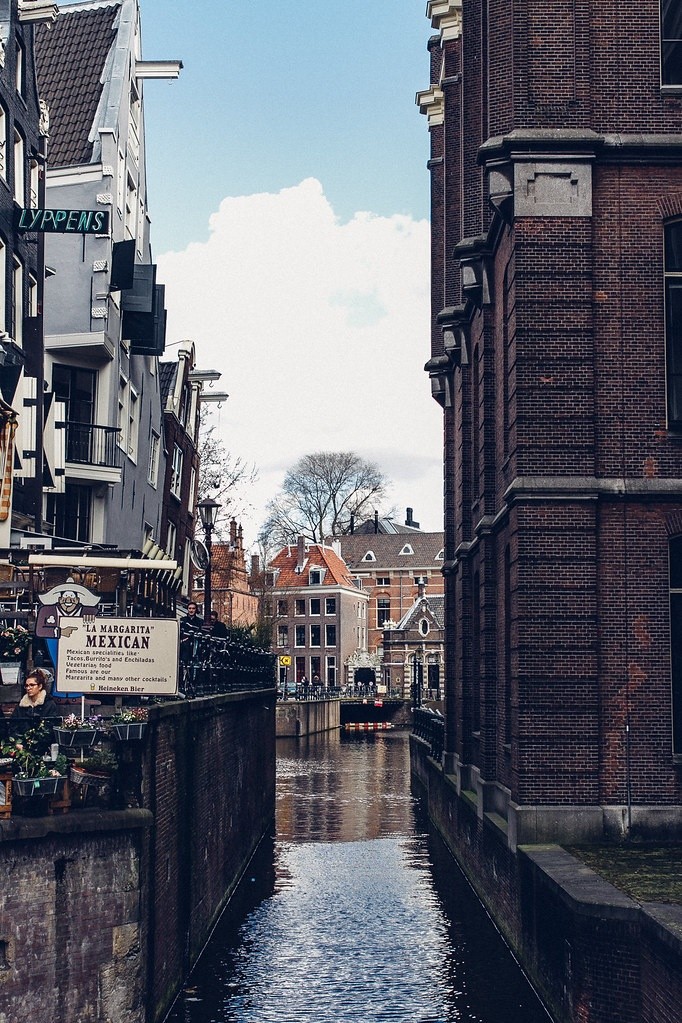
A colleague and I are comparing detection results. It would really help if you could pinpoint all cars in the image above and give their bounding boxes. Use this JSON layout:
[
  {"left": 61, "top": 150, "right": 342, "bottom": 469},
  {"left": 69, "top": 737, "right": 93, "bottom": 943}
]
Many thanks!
[{"left": 277, "top": 684, "right": 299, "bottom": 695}]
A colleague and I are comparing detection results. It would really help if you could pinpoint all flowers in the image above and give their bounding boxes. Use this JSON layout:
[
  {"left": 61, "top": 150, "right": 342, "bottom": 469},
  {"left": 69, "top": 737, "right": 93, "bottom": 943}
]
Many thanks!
[
  {"left": 0, "top": 625, "right": 33, "bottom": 661},
  {"left": 109, "top": 708, "right": 148, "bottom": 725},
  {"left": 0, "top": 722, "right": 53, "bottom": 777},
  {"left": 59, "top": 712, "right": 106, "bottom": 730}
]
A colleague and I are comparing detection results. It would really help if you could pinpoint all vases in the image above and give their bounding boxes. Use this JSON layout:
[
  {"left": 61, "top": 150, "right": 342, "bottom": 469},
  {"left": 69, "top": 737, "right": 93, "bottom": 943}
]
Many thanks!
[
  {"left": 9, "top": 774, "right": 68, "bottom": 796},
  {"left": 111, "top": 722, "right": 146, "bottom": 740},
  {"left": 0, "top": 662, "right": 22, "bottom": 685},
  {"left": 53, "top": 726, "right": 105, "bottom": 746}
]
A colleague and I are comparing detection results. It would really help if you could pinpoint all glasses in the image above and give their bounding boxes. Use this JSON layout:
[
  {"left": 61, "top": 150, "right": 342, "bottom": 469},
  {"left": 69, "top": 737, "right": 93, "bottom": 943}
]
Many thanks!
[{"left": 23, "top": 683, "right": 39, "bottom": 689}]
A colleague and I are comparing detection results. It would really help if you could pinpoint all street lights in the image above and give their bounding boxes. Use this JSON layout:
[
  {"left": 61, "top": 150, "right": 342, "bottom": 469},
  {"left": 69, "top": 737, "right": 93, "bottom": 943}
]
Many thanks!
[
  {"left": 282, "top": 644, "right": 291, "bottom": 701},
  {"left": 412, "top": 651, "right": 418, "bottom": 708},
  {"left": 414, "top": 646, "right": 424, "bottom": 709},
  {"left": 195, "top": 495, "right": 223, "bottom": 629}
]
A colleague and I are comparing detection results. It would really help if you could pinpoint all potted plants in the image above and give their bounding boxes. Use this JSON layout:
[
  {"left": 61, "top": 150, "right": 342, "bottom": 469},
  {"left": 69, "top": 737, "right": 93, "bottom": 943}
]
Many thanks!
[{"left": 70, "top": 740, "right": 118, "bottom": 787}]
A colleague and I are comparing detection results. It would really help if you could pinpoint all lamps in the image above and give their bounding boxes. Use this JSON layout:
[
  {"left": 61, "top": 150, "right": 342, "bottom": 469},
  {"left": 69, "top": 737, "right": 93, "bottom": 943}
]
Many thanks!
[
  {"left": 179, "top": 693, "right": 186, "bottom": 699},
  {"left": 140, "top": 696, "right": 148, "bottom": 702},
  {"left": 141, "top": 537, "right": 182, "bottom": 586}
]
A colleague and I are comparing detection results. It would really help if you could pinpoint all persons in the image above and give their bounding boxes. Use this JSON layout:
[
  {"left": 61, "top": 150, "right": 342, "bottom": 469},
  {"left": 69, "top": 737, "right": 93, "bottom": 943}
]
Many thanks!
[
  {"left": 313, "top": 676, "right": 324, "bottom": 686},
  {"left": 301, "top": 676, "right": 309, "bottom": 686},
  {"left": 180, "top": 602, "right": 203, "bottom": 630},
  {"left": 211, "top": 610, "right": 226, "bottom": 637},
  {"left": 8, "top": 669, "right": 58, "bottom": 778}
]
